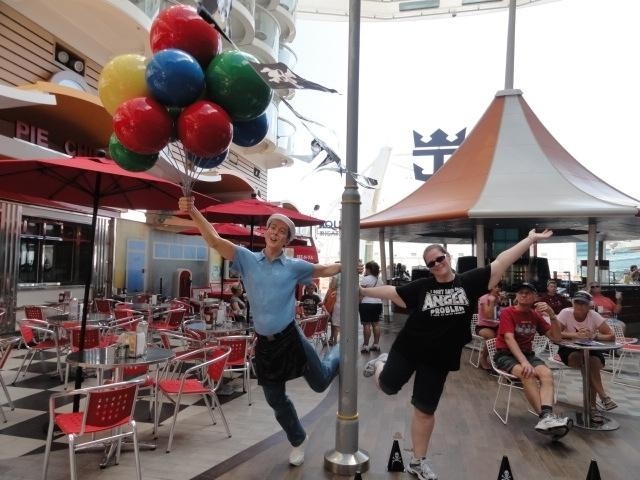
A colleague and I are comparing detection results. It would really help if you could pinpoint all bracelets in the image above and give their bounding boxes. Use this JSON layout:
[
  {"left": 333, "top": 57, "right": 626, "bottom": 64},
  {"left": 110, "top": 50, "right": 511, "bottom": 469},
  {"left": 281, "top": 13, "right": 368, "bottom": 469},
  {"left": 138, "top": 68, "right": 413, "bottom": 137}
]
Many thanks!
[{"left": 550, "top": 313, "right": 556, "bottom": 320}]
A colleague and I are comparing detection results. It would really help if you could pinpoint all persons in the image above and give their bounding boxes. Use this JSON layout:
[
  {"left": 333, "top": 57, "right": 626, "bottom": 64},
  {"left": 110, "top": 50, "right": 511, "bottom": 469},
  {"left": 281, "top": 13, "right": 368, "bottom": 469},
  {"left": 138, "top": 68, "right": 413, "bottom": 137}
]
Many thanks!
[
  {"left": 177, "top": 193, "right": 342, "bottom": 471},
  {"left": 359, "top": 227, "right": 556, "bottom": 479},
  {"left": 230, "top": 282, "right": 245, "bottom": 322},
  {"left": 555, "top": 289, "right": 619, "bottom": 414},
  {"left": 299, "top": 283, "right": 328, "bottom": 321},
  {"left": 358, "top": 261, "right": 384, "bottom": 352},
  {"left": 540, "top": 262, "right": 640, "bottom": 337},
  {"left": 476, "top": 282, "right": 499, "bottom": 370},
  {"left": 325, "top": 261, "right": 342, "bottom": 346},
  {"left": 494, "top": 282, "right": 573, "bottom": 439}
]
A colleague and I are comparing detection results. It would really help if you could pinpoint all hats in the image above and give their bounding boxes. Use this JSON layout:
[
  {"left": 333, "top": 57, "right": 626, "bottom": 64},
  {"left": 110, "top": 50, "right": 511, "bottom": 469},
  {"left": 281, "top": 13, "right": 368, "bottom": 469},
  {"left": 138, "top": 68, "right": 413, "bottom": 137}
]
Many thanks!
[
  {"left": 516, "top": 281, "right": 536, "bottom": 295},
  {"left": 266, "top": 212, "right": 296, "bottom": 244},
  {"left": 572, "top": 290, "right": 591, "bottom": 305}
]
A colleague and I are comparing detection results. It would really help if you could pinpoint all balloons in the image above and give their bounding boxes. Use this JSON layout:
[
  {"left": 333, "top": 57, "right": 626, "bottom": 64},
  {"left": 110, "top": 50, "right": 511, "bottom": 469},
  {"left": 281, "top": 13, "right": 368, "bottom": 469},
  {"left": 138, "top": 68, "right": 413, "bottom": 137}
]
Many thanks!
[{"left": 98, "top": 3, "right": 272, "bottom": 174}]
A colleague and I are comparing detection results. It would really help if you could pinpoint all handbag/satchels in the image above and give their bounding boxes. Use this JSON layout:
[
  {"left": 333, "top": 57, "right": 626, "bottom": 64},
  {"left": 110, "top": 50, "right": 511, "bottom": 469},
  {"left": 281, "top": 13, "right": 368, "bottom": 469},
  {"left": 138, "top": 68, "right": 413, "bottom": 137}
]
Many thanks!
[{"left": 323, "top": 288, "right": 338, "bottom": 314}]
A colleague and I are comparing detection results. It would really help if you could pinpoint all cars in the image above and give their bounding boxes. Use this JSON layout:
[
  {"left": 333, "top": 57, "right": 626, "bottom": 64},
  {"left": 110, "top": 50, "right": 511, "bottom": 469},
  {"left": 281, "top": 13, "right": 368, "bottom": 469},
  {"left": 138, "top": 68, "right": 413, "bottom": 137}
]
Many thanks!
[{"left": 553, "top": 279, "right": 640, "bottom": 339}]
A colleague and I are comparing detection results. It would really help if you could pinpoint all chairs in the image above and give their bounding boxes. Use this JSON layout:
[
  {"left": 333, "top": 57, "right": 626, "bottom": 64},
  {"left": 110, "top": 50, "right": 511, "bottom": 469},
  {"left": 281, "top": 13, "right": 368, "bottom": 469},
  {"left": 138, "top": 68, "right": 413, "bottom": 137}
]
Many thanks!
[
  {"left": 42, "top": 380, "right": 142, "bottom": 480},
  {"left": 469, "top": 290, "right": 640, "bottom": 423},
  {"left": 0, "top": 277, "right": 329, "bottom": 426},
  {"left": 152, "top": 347, "right": 232, "bottom": 453}
]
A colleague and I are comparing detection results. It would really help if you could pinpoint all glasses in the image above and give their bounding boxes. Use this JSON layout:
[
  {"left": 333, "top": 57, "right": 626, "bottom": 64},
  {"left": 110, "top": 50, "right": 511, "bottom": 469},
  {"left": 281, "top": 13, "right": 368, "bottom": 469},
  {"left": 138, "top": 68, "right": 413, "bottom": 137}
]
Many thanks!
[
  {"left": 427, "top": 253, "right": 447, "bottom": 268},
  {"left": 591, "top": 285, "right": 601, "bottom": 289}
]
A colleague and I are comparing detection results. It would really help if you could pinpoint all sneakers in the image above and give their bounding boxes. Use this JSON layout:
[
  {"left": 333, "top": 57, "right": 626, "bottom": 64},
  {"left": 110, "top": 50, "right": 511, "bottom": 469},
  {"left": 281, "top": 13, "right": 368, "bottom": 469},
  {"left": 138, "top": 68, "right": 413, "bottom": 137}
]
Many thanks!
[
  {"left": 534, "top": 411, "right": 574, "bottom": 441},
  {"left": 359, "top": 341, "right": 381, "bottom": 353},
  {"left": 362, "top": 352, "right": 389, "bottom": 378},
  {"left": 287, "top": 434, "right": 310, "bottom": 466},
  {"left": 407, "top": 456, "right": 438, "bottom": 480},
  {"left": 590, "top": 407, "right": 604, "bottom": 427}
]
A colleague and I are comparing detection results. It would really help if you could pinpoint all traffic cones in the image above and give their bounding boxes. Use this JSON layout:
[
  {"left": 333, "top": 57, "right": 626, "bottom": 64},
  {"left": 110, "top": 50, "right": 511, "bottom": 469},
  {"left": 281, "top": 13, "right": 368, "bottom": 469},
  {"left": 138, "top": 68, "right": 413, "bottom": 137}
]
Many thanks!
[
  {"left": 586, "top": 458, "right": 600, "bottom": 480},
  {"left": 387, "top": 439, "right": 408, "bottom": 475},
  {"left": 497, "top": 453, "right": 514, "bottom": 480}
]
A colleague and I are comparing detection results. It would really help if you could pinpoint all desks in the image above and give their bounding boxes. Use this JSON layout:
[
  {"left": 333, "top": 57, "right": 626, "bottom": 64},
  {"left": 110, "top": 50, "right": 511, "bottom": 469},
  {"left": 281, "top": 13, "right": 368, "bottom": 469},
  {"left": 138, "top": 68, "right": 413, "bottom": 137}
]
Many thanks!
[
  {"left": 555, "top": 338, "right": 624, "bottom": 432},
  {"left": 65, "top": 346, "right": 176, "bottom": 444}
]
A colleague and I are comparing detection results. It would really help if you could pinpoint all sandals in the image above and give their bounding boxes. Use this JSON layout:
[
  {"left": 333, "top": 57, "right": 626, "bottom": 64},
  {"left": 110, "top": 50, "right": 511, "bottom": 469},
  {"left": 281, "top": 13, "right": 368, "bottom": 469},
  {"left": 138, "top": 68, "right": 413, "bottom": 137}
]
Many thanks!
[{"left": 603, "top": 396, "right": 618, "bottom": 411}]
